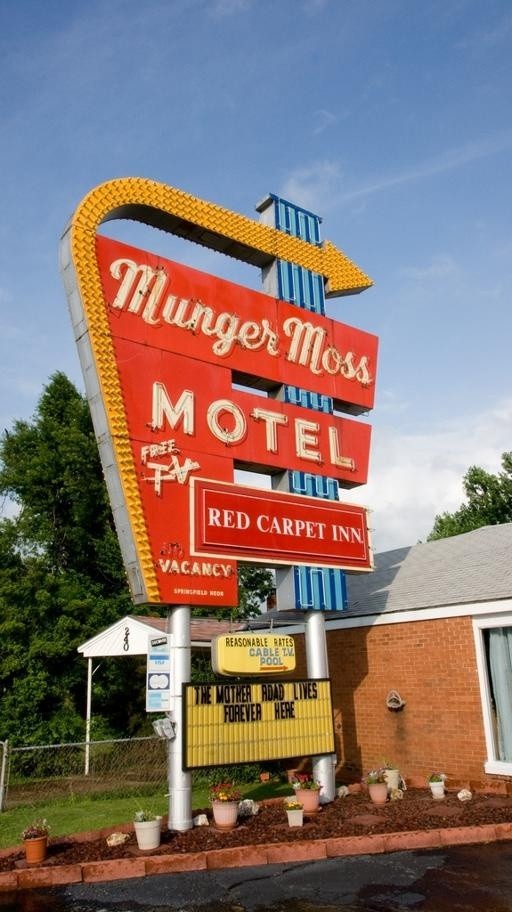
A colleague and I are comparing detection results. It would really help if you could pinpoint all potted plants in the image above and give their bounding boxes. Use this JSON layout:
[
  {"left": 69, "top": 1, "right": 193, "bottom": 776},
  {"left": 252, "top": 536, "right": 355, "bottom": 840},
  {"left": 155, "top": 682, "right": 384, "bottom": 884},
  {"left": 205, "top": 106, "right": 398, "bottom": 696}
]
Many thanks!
[
  {"left": 293, "top": 777, "right": 324, "bottom": 814},
  {"left": 133, "top": 791, "right": 164, "bottom": 851}
]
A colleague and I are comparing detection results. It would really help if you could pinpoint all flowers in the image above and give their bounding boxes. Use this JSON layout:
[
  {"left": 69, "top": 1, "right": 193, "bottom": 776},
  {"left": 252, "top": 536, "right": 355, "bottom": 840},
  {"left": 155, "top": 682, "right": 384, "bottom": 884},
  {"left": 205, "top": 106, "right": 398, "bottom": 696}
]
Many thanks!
[
  {"left": 284, "top": 802, "right": 304, "bottom": 810},
  {"left": 21, "top": 819, "right": 51, "bottom": 839},
  {"left": 429, "top": 771, "right": 442, "bottom": 782},
  {"left": 366, "top": 768, "right": 389, "bottom": 785},
  {"left": 209, "top": 783, "right": 242, "bottom": 801}
]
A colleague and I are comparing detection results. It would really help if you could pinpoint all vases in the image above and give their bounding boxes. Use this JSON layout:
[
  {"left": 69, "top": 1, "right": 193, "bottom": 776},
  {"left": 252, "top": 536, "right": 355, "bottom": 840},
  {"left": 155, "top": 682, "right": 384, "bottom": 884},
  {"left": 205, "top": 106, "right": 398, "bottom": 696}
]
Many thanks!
[
  {"left": 383, "top": 769, "right": 400, "bottom": 789},
  {"left": 429, "top": 781, "right": 445, "bottom": 799},
  {"left": 211, "top": 799, "right": 241, "bottom": 829},
  {"left": 23, "top": 835, "right": 48, "bottom": 864},
  {"left": 286, "top": 810, "right": 304, "bottom": 827},
  {"left": 369, "top": 782, "right": 388, "bottom": 804}
]
